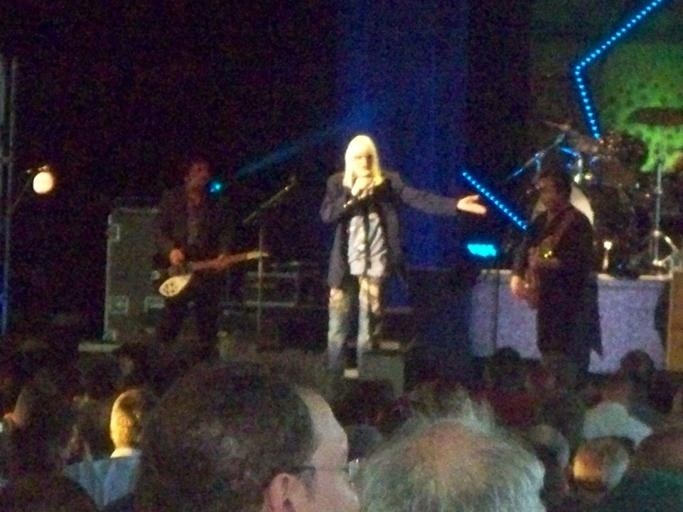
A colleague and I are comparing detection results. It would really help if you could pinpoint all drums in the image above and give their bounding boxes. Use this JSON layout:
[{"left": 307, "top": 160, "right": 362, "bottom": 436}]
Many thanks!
[
  {"left": 591, "top": 132, "right": 648, "bottom": 180},
  {"left": 531, "top": 182, "right": 639, "bottom": 270}
]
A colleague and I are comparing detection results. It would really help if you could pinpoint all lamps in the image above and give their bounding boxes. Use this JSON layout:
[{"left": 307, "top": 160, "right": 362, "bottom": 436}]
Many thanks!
[{"left": 7, "top": 158, "right": 56, "bottom": 231}]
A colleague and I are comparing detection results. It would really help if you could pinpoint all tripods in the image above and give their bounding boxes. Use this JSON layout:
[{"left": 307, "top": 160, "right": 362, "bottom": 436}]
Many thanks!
[{"left": 631, "top": 165, "right": 679, "bottom": 261}]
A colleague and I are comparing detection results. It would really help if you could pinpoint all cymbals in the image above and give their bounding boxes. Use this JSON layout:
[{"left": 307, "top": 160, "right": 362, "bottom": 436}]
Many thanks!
[
  {"left": 545, "top": 119, "right": 588, "bottom": 144},
  {"left": 627, "top": 107, "right": 683, "bottom": 126}
]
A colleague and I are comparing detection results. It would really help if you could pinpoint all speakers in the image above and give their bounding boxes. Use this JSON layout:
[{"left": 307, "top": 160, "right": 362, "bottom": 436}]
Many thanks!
[
  {"left": 101, "top": 206, "right": 166, "bottom": 343},
  {"left": 358, "top": 344, "right": 469, "bottom": 397}
]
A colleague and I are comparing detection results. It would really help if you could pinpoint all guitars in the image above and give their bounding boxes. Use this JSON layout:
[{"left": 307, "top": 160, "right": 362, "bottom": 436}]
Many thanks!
[
  {"left": 151, "top": 243, "right": 268, "bottom": 300},
  {"left": 521, "top": 237, "right": 553, "bottom": 307}
]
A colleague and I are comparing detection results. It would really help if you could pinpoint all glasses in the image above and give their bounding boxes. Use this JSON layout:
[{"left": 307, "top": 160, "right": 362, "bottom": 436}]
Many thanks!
[{"left": 279, "top": 459, "right": 361, "bottom": 485}]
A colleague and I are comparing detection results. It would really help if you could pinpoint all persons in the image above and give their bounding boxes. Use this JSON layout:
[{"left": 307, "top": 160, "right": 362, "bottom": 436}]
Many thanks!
[
  {"left": 318, "top": 133, "right": 487, "bottom": 376},
  {"left": 0, "top": 318, "right": 682, "bottom": 512},
  {"left": 155, "top": 153, "right": 237, "bottom": 341},
  {"left": 510, "top": 168, "right": 599, "bottom": 401}
]
